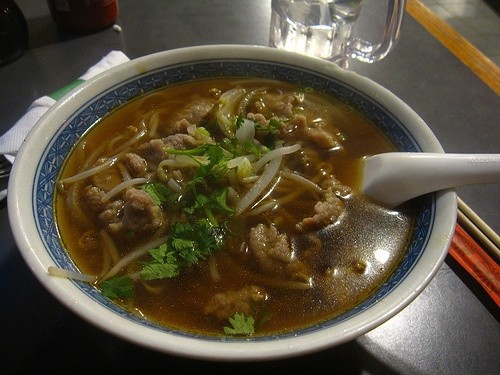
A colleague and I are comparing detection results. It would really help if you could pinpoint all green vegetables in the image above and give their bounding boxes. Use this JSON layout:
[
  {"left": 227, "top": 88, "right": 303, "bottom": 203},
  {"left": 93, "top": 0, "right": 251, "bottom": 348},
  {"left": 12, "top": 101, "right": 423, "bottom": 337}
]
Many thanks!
[{"left": 172, "top": 141, "right": 226, "bottom": 265}]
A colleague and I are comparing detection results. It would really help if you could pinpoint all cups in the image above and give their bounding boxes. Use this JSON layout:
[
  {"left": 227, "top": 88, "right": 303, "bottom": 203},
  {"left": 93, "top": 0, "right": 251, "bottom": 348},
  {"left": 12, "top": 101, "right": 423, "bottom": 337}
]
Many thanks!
[{"left": 267, "top": 0, "right": 407, "bottom": 70}]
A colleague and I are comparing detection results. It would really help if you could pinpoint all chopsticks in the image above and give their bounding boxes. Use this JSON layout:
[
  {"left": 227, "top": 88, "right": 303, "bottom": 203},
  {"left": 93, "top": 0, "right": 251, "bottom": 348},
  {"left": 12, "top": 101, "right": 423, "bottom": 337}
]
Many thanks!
[{"left": 456, "top": 196, "right": 500, "bottom": 260}]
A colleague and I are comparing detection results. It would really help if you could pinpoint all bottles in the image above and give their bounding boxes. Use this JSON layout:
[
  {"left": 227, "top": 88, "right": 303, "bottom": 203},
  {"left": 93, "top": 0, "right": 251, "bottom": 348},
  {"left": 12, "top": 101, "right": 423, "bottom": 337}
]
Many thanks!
[
  {"left": 47, "top": 0, "right": 120, "bottom": 35},
  {"left": 0, "top": 0, "right": 29, "bottom": 68}
]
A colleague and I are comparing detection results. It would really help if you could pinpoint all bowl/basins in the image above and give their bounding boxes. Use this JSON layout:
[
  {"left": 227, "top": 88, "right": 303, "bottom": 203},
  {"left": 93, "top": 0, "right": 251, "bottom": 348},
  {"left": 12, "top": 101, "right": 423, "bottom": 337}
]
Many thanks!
[{"left": 6, "top": 43, "right": 457, "bottom": 360}]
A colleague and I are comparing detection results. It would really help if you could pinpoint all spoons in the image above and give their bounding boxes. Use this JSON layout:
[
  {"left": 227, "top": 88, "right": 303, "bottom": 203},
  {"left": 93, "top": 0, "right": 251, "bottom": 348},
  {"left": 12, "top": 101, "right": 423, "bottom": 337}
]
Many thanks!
[{"left": 360, "top": 152, "right": 500, "bottom": 208}]
